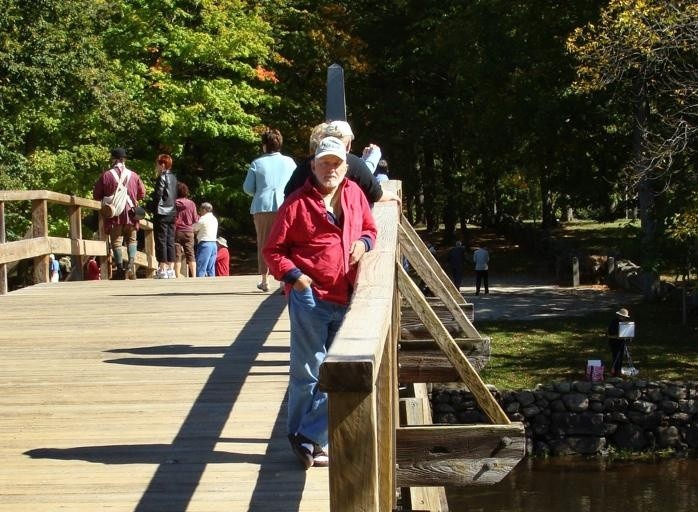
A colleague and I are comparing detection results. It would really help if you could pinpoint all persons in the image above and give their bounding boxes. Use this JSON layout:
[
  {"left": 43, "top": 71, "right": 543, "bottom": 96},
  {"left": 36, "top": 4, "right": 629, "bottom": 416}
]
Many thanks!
[
  {"left": 606, "top": 308, "right": 630, "bottom": 377},
  {"left": 429, "top": 238, "right": 489, "bottom": 294},
  {"left": 193, "top": 202, "right": 219, "bottom": 276},
  {"left": 216, "top": 236, "right": 230, "bottom": 276},
  {"left": 242, "top": 131, "right": 298, "bottom": 291},
  {"left": 93, "top": 147, "right": 145, "bottom": 279},
  {"left": 176, "top": 183, "right": 200, "bottom": 276},
  {"left": 261, "top": 136, "right": 377, "bottom": 470},
  {"left": 283, "top": 121, "right": 383, "bottom": 207},
  {"left": 87, "top": 256, "right": 99, "bottom": 279},
  {"left": 143, "top": 154, "right": 180, "bottom": 278},
  {"left": 48, "top": 254, "right": 60, "bottom": 283}
]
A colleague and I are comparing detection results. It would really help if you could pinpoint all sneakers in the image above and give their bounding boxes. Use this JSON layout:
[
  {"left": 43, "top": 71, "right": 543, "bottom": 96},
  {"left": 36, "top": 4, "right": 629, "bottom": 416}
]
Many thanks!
[
  {"left": 154, "top": 270, "right": 176, "bottom": 279},
  {"left": 287, "top": 431, "right": 329, "bottom": 469},
  {"left": 108, "top": 264, "right": 136, "bottom": 280},
  {"left": 257, "top": 281, "right": 269, "bottom": 292},
  {"left": 279, "top": 286, "right": 285, "bottom": 294}
]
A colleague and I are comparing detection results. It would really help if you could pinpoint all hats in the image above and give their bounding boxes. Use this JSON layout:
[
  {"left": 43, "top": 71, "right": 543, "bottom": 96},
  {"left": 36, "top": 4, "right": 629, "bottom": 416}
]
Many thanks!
[
  {"left": 109, "top": 148, "right": 132, "bottom": 161},
  {"left": 325, "top": 121, "right": 355, "bottom": 140},
  {"left": 216, "top": 237, "right": 229, "bottom": 248},
  {"left": 315, "top": 135, "right": 346, "bottom": 161},
  {"left": 616, "top": 308, "right": 630, "bottom": 318}
]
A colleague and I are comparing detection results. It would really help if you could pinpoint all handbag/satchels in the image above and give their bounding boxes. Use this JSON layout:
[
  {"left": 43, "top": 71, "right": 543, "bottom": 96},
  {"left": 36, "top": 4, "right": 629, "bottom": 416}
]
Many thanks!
[
  {"left": 586, "top": 365, "right": 605, "bottom": 383},
  {"left": 101, "top": 188, "right": 145, "bottom": 220}
]
